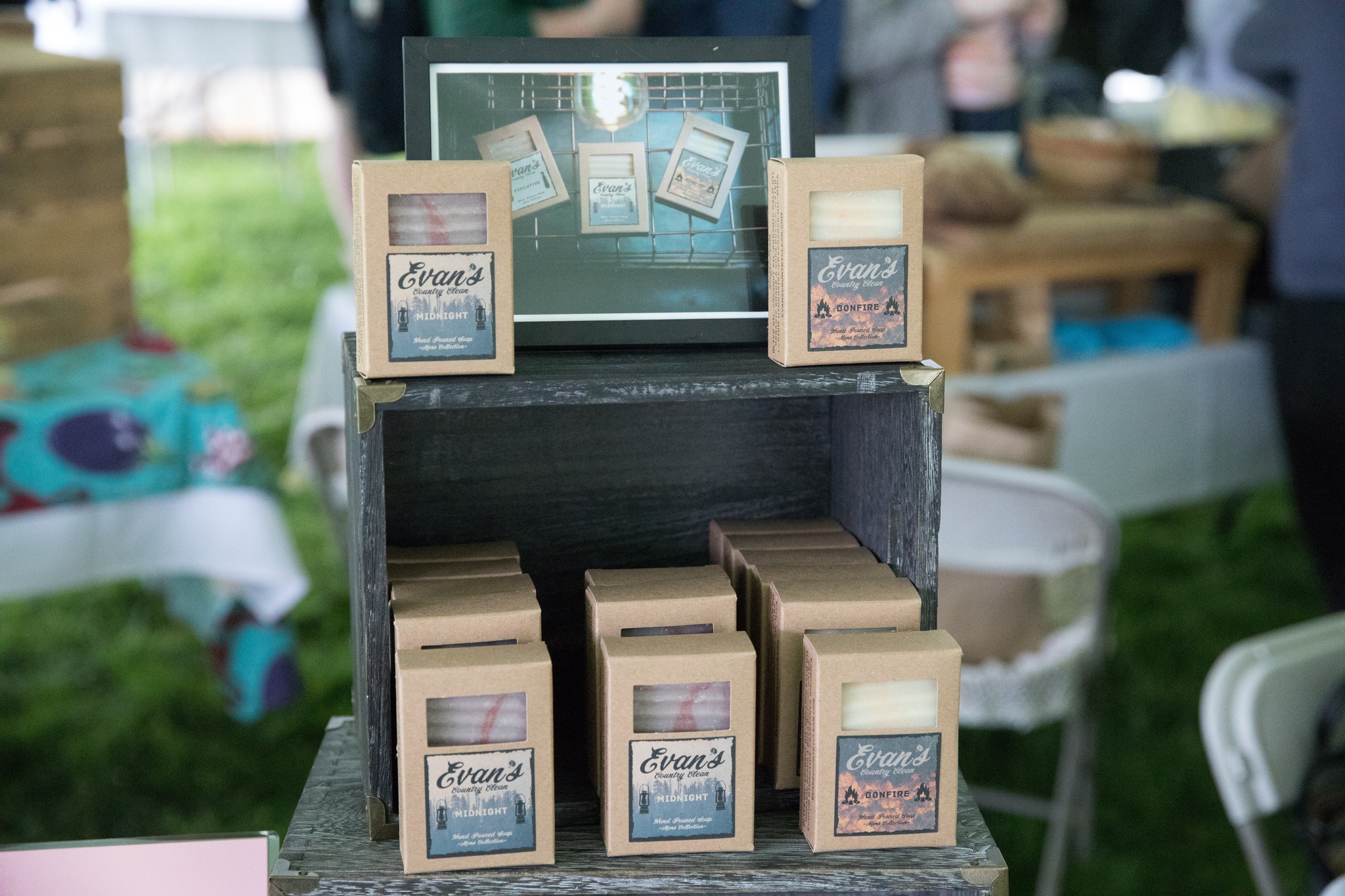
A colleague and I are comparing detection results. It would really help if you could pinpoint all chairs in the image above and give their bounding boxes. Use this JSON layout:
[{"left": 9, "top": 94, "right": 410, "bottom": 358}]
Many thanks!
[
  {"left": 935, "top": 453, "right": 1116, "bottom": 896},
  {"left": 1199, "top": 611, "right": 1345, "bottom": 896}
]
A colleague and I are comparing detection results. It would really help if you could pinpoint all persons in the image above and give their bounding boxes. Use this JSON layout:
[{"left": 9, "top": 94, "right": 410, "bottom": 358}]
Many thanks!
[
  {"left": 1233, "top": 0, "right": 1345, "bottom": 613},
  {"left": 305, "top": 0, "right": 1193, "bottom": 239}
]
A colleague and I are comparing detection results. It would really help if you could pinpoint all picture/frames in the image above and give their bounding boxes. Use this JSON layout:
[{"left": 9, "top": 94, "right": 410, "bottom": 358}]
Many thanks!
[{"left": 402, "top": 37, "right": 822, "bottom": 349}]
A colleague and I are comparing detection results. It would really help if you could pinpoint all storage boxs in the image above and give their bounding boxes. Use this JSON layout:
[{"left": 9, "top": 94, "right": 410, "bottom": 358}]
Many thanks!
[
  {"left": 474, "top": 115, "right": 571, "bottom": 221},
  {"left": 655, "top": 111, "right": 751, "bottom": 221},
  {"left": 762, "top": 150, "right": 926, "bottom": 366},
  {"left": 576, "top": 141, "right": 650, "bottom": 238},
  {"left": 390, "top": 518, "right": 962, "bottom": 880},
  {"left": 350, "top": 157, "right": 515, "bottom": 376}
]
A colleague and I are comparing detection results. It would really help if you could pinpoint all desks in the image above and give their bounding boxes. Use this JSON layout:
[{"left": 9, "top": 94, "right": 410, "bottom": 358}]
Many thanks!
[{"left": 923, "top": 185, "right": 1286, "bottom": 520}]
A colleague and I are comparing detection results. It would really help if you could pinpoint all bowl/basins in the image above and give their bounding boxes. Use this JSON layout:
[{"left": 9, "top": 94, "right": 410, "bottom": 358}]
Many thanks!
[{"left": 1022, "top": 117, "right": 1159, "bottom": 198}]
